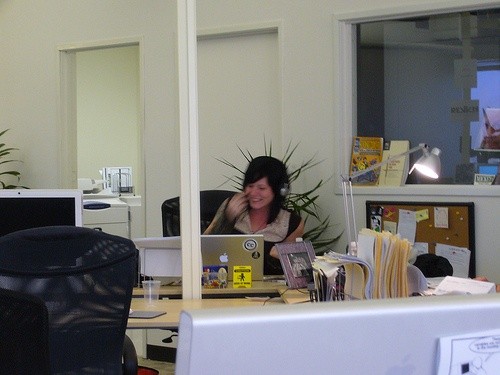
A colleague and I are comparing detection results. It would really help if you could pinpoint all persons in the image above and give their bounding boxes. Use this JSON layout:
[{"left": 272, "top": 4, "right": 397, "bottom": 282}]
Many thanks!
[{"left": 202, "top": 156, "right": 305, "bottom": 275}]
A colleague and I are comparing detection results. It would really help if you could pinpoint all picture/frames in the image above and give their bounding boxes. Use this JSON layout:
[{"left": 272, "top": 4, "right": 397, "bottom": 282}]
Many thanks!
[{"left": 276, "top": 240, "right": 316, "bottom": 289}]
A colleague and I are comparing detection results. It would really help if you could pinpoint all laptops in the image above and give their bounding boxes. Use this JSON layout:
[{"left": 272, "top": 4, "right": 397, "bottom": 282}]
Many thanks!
[{"left": 200, "top": 234, "right": 264, "bottom": 281}]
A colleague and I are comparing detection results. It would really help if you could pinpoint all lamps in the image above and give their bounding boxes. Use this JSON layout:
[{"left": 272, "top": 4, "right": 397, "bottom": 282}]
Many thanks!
[{"left": 342, "top": 143, "right": 441, "bottom": 258}]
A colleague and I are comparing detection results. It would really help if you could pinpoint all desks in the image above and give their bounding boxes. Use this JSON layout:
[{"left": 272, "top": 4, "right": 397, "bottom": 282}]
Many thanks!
[
  {"left": 127, "top": 299, "right": 283, "bottom": 328},
  {"left": 132, "top": 275, "right": 285, "bottom": 298}
]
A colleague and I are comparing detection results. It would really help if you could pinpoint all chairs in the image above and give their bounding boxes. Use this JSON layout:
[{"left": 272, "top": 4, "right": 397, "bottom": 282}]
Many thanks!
[
  {"left": 161, "top": 190, "right": 240, "bottom": 343},
  {"left": 0, "top": 226, "right": 138, "bottom": 375}
]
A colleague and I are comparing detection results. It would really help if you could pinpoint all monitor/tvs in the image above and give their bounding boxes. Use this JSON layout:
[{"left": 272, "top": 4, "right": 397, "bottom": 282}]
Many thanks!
[
  {"left": 175, "top": 293, "right": 500, "bottom": 375},
  {"left": 0, "top": 188, "right": 83, "bottom": 238}
]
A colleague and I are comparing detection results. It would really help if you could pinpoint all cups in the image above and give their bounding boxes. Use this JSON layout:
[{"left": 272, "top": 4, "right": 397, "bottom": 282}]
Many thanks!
[{"left": 142, "top": 280, "right": 161, "bottom": 307}]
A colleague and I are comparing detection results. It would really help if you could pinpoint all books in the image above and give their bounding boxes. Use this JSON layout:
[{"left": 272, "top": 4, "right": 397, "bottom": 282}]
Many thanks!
[{"left": 349, "top": 136, "right": 411, "bottom": 187}]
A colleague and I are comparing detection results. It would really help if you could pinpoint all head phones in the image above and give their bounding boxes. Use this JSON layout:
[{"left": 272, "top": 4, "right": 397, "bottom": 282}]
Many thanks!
[{"left": 278, "top": 165, "right": 290, "bottom": 197}]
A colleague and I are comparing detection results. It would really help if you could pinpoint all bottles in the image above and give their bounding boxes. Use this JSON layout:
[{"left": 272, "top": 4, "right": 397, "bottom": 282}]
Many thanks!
[{"left": 346, "top": 241, "right": 357, "bottom": 256}]
